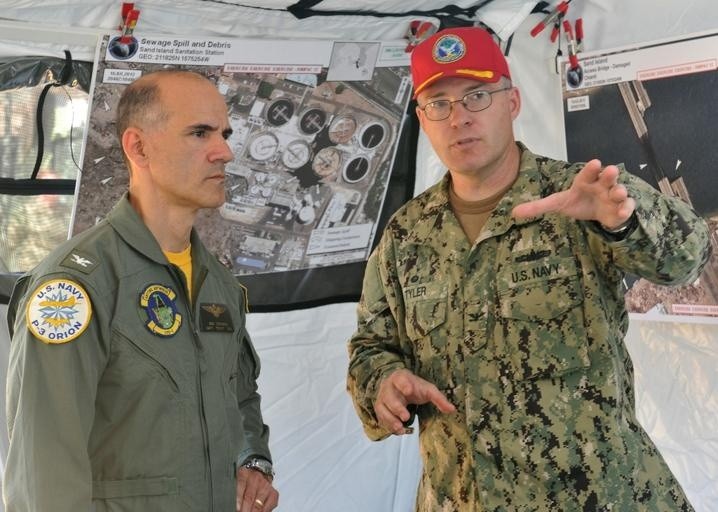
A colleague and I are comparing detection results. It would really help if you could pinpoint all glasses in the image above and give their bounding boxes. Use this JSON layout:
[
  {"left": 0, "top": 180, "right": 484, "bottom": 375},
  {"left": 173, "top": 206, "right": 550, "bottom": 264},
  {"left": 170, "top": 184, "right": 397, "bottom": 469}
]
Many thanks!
[{"left": 418, "top": 87, "right": 512, "bottom": 121}]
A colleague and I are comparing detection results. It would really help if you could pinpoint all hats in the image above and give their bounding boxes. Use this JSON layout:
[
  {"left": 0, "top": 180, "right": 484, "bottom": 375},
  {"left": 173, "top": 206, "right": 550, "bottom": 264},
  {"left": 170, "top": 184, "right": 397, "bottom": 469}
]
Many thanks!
[{"left": 411, "top": 26, "right": 511, "bottom": 100}]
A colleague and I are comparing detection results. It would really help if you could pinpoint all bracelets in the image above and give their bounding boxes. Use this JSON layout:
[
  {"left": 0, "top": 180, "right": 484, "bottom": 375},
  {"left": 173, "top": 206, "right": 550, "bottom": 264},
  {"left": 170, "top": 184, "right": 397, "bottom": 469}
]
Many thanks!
[{"left": 600, "top": 217, "right": 633, "bottom": 236}]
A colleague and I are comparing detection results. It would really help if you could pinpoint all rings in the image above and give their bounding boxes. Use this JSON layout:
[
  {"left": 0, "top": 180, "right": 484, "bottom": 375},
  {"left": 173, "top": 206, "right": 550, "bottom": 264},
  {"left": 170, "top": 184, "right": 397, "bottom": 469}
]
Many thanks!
[{"left": 256, "top": 499, "right": 264, "bottom": 507}]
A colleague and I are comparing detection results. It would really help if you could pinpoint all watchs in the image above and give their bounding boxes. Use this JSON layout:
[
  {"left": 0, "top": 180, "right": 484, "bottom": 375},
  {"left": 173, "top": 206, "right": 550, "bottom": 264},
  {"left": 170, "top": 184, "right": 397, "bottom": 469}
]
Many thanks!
[{"left": 244, "top": 457, "right": 275, "bottom": 479}]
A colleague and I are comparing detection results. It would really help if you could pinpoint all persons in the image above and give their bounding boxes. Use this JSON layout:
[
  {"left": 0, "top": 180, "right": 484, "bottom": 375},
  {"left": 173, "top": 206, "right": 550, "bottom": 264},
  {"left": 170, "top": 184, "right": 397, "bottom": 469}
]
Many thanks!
[
  {"left": 1, "top": 69, "right": 279, "bottom": 512},
  {"left": 345, "top": 26, "right": 712, "bottom": 512}
]
